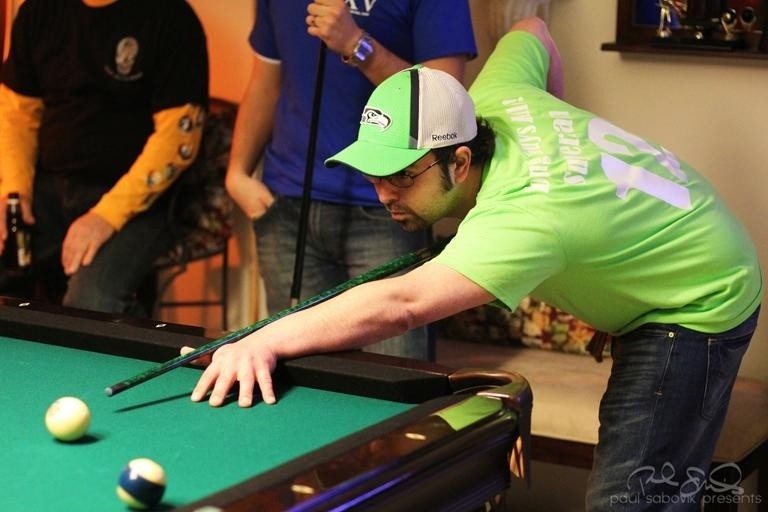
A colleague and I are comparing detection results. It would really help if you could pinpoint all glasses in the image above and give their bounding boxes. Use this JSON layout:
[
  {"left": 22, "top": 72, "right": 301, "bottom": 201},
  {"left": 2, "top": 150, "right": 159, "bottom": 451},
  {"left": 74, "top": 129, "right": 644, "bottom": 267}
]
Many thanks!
[{"left": 360, "top": 158, "right": 438, "bottom": 187}]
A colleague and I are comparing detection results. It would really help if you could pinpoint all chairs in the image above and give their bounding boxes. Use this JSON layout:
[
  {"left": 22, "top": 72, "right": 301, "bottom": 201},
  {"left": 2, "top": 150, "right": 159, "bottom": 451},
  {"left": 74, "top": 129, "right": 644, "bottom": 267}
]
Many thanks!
[{"left": 142, "top": 92, "right": 245, "bottom": 333}]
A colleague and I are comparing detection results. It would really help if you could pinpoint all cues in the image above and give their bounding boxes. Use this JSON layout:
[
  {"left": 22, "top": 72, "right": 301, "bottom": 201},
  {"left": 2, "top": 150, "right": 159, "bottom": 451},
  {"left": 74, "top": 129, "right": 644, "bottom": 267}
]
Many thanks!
[
  {"left": 290, "top": 38, "right": 327, "bottom": 313},
  {"left": 103, "top": 235, "right": 457, "bottom": 396}
]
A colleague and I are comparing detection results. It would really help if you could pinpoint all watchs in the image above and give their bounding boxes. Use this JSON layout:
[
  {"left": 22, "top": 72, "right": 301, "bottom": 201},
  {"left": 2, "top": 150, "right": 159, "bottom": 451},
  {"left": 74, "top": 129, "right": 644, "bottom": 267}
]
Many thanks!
[{"left": 340, "top": 32, "right": 377, "bottom": 68}]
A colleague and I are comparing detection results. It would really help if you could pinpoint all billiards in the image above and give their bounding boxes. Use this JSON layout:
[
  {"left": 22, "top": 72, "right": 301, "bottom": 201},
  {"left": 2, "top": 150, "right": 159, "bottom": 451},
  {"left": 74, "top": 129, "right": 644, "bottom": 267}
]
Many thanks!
[
  {"left": 116, "top": 458, "right": 166, "bottom": 508},
  {"left": 46, "top": 397, "right": 90, "bottom": 441}
]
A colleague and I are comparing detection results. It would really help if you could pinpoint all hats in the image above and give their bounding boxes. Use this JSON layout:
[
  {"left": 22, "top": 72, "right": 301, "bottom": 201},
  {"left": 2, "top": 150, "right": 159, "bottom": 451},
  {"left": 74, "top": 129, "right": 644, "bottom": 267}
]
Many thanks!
[{"left": 324, "top": 63, "right": 478, "bottom": 177}]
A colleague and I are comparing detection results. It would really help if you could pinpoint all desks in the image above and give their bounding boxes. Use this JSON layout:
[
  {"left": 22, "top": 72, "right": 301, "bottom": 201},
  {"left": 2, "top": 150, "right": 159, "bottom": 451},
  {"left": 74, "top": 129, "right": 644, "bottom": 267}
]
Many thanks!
[{"left": 0, "top": 291, "right": 536, "bottom": 508}]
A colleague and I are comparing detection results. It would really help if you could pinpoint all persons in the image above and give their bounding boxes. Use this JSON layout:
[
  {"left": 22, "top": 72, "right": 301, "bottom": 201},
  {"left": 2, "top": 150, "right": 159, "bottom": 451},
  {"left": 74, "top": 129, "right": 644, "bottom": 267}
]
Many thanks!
[
  {"left": 180, "top": 16, "right": 763, "bottom": 512},
  {"left": 0, "top": 0, "right": 209, "bottom": 318},
  {"left": 224, "top": 0, "right": 477, "bottom": 362}
]
